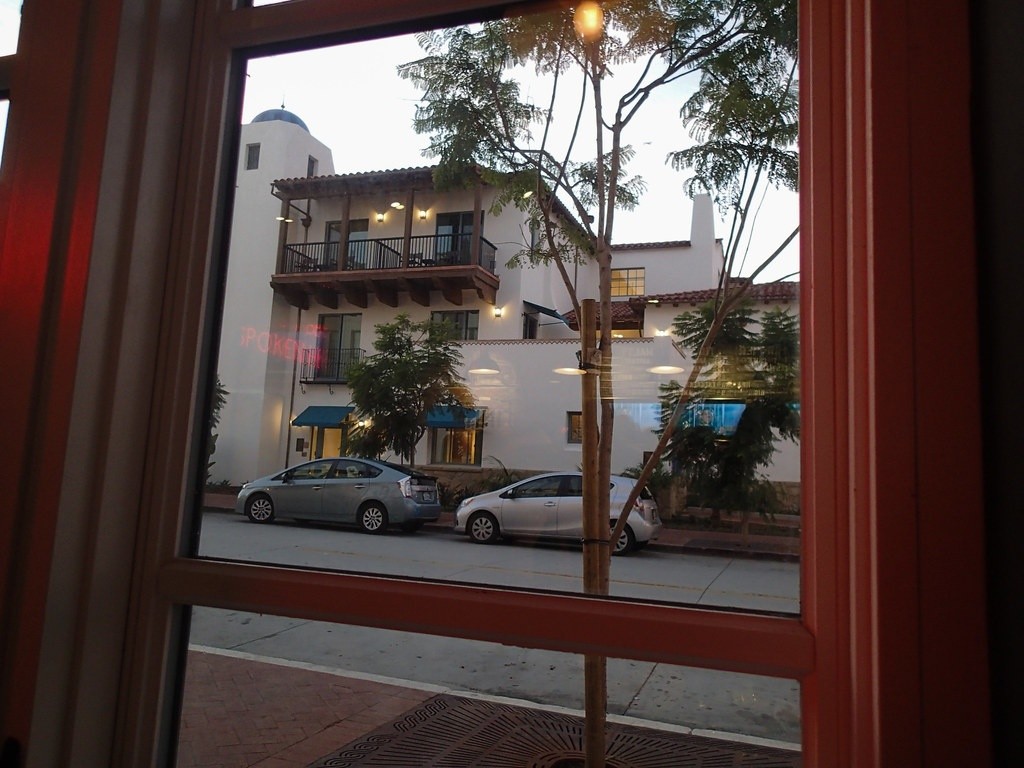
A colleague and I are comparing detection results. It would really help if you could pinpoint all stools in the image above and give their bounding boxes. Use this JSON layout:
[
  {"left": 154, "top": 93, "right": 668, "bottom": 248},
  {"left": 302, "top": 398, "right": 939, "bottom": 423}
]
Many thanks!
[{"left": 422, "top": 259, "right": 435, "bottom": 266}]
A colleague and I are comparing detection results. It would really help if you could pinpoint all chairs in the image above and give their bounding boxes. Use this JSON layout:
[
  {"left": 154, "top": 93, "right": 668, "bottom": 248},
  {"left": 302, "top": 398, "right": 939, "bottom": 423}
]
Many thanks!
[
  {"left": 400, "top": 252, "right": 423, "bottom": 267},
  {"left": 300, "top": 255, "right": 365, "bottom": 272},
  {"left": 337, "top": 467, "right": 348, "bottom": 478}
]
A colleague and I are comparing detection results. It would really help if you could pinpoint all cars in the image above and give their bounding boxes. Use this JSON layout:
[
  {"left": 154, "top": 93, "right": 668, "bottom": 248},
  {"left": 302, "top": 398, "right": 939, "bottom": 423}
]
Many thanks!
[
  {"left": 235, "top": 456, "right": 443, "bottom": 536},
  {"left": 456, "top": 472, "right": 663, "bottom": 556}
]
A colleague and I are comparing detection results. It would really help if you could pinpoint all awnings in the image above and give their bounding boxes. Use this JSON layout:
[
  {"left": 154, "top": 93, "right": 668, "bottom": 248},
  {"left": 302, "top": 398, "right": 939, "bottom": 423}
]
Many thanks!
[
  {"left": 523, "top": 300, "right": 569, "bottom": 323},
  {"left": 292, "top": 406, "right": 355, "bottom": 427},
  {"left": 423, "top": 406, "right": 479, "bottom": 428}
]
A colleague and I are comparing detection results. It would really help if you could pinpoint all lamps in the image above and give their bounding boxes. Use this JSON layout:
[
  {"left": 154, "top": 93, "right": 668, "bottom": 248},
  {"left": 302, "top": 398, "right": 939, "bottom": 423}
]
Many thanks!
[
  {"left": 420, "top": 210, "right": 426, "bottom": 219},
  {"left": 553, "top": 353, "right": 588, "bottom": 375},
  {"left": 494, "top": 307, "right": 501, "bottom": 317},
  {"left": 646, "top": 346, "right": 685, "bottom": 375},
  {"left": 359, "top": 420, "right": 364, "bottom": 428},
  {"left": 377, "top": 212, "right": 384, "bottom": 222},
  {"left": 468, "top": 349, "right": 501, "bottom": 375}
]
